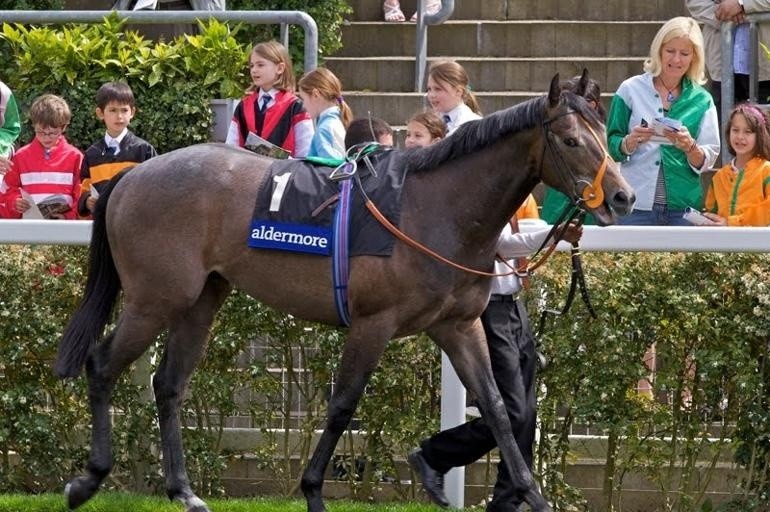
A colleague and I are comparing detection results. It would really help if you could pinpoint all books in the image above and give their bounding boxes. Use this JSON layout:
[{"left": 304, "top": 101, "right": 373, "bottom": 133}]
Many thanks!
[
  {"left": 20, "top": 186, "right": 74, "bottom": 220},
  {"left": 242, "top": 131, "right": 293, "bottom": 160},
  {"left": 639, "top": 115, "right": 681, "bottom": 145},
  {"left": 682, "top": 205, "right": 716, "bottom": 227}
]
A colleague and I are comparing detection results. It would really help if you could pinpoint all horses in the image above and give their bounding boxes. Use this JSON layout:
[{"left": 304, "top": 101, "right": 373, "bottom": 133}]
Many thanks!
[{"left": 52, "top": 67, "right": 637, "bottom": 512}]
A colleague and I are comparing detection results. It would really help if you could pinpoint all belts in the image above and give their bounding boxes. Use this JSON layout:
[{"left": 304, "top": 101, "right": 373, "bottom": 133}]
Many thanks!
[{"left": 491, "top": 292, "right": 520, "bottom": 301}]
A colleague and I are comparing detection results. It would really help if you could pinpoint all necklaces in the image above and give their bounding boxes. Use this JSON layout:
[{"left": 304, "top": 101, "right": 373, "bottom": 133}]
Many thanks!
[{"left": 657, "top": 74, "right": 681, "bottom": 103}]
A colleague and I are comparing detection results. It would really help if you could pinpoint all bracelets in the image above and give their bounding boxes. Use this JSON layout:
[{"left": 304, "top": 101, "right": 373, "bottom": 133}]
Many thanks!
[{"left": 685, "top": 139, "right": 696, "bottom": 155}]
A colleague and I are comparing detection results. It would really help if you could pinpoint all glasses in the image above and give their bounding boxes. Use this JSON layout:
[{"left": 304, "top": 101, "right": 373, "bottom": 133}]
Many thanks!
[{"left": 35, "top": 127, "right": 62, "bottom": 136}]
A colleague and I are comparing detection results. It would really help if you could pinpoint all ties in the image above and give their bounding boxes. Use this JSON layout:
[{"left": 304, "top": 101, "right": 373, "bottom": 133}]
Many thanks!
[{"left": 256, "top": 94, "right": 272, "bottom": 139}]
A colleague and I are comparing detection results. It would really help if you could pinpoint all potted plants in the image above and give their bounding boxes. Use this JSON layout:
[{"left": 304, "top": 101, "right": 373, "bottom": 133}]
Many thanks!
[{"left": 208, "top": 36, "right": 251, "bottom": 143}]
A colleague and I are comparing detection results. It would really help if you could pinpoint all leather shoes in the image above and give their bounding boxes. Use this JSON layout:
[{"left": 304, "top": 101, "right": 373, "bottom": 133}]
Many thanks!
[{"left": 406, "top": 447, "right": 450, "bottom": 510}]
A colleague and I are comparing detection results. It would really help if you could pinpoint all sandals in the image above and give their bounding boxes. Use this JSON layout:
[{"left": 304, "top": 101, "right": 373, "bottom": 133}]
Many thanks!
[
  {"left": 410, "top": 2, "right": 443, "bottom": 23},
  {"left": 382, "top": 1, "right": 407, "bottom": 22}
]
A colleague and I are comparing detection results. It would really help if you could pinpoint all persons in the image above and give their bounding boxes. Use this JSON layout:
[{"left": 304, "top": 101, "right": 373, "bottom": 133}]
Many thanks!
[
  {"left": 686, "top": 1, "right": 770, "bottom": 125},
  {"left": 410, "top": 220, "right": 583, "bottom": 512},
  {"left": 2, "top": 156, "right": 15, "bottom": 179},
  {"left": 380, "top": 0, "right": 445, "bottom": 24},
  {"left": 0, "top": 74, "right": 21, "bottom": 156},
  {"left": 345, "top": 117, "right": 395, "bottom": 156},
  {"left": 541, "top": 75, "right": 609, "bottom": 226},
  {"left": 515, "top": 193, "right": 539, "bottom": 219},
  {"left": 223, "top": 41, "right": 314, "bottom": 159},
  {"left": 606, "top": 16, "right": 722, "bottom": 226},
  {"left": 683, "top": 102, "right": 770, "bottom": 227},
  {"left": 404, "top": 114, "right": 446, "bottom": 147},
  {"left": 78, "top": 80, "right": 160, "bottom": 221},
  {"left": 0, "top": 92, "right": 88, "bottom": 221},
  {"left": 426, "top": 61, "right": 483, "bottom": 142},
  {"left": 297, "top": 68, "right": 355, "bottom": 163}
]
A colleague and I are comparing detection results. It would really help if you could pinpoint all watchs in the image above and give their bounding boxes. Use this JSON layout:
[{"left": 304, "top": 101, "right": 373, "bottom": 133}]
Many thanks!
[{"left": 739, "top": 0, "right": 744, "bottom": 12}]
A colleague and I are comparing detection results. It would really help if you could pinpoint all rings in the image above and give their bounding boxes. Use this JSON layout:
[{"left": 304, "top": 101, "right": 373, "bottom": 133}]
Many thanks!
[{"left": 635, "top": 137, "right": 642, "bottom": 144}]
